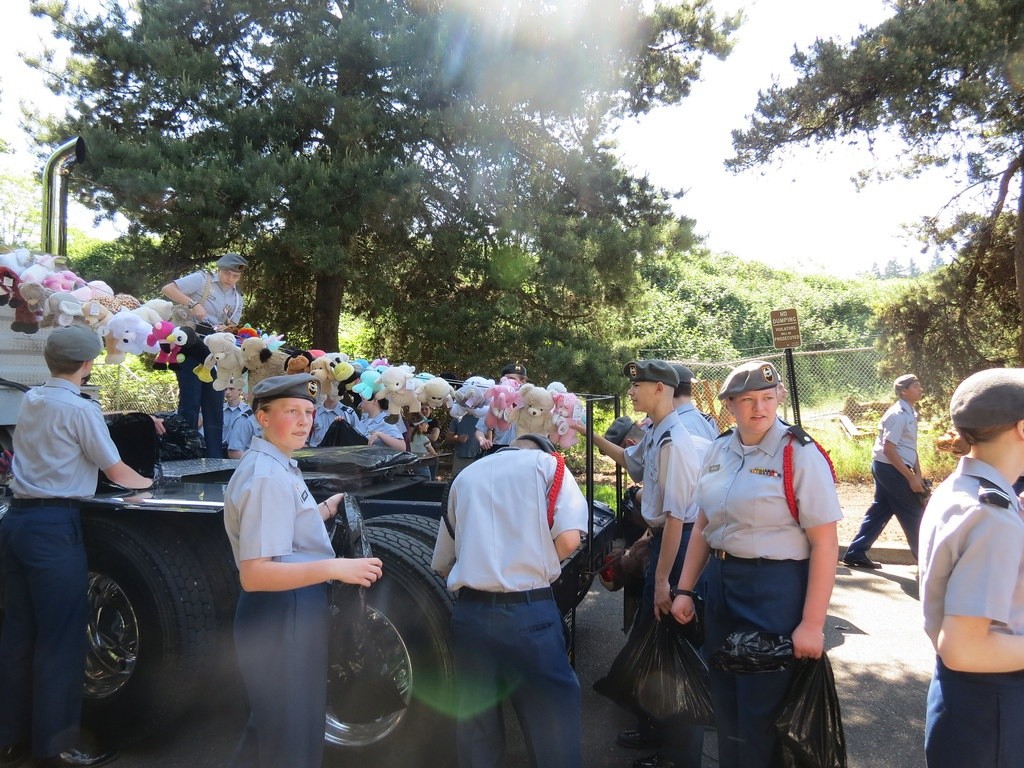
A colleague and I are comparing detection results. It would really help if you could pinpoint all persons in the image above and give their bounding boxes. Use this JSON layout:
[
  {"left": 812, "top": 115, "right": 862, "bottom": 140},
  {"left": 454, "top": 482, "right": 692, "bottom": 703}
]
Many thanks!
[
  {"left": 431, "top": 434, "right": 584, "bottom": 768},
  {"left": 917, "top": 367, "right": 1024, "bottom": 767},
  {"left": 223, "top": 373, "right": 383, "bottom": 767},
  {"left": 842, "top": 375, "right": 928, "bottom": 569},
  {"left": 777, "top": 373, "right": 788, "bottom": 402},
  {"left": 568, "top": 360, "right": 842, "bottom": 768},
  {"left": 161, "top": 254, "right": 248, "bottom": 459},
  {"left": 0, "top": 324, "right": 166, "bottom": 768},
  {"left": 224, "top": 362, "right": 529, "bottom": 481}
]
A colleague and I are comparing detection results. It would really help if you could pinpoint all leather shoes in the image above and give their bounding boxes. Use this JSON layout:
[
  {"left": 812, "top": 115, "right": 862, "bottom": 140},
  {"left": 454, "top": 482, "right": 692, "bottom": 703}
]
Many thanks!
[
  {"left": 616, "top": 730, "right": 659, "bottom": 748},
  {"left": 631, "top": 754, "right": 685, "bottom": 768},
  {"left": 32, "top": 743, "right": 121, "bottom": 768},
  {"left": 0, "top": 727, "right": 33, "bottom": 768},
  {"left": 843, "top": 558, "right": 883, "bottom": 569}
]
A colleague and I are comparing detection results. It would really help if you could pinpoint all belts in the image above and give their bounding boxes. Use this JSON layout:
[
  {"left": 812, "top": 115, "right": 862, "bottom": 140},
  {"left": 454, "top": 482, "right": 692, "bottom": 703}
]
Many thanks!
[
  {"left": 707, "top": 546, "right": 765, "bottom": 563},
  {"left": 10, "top": 498, "right": 79, "bottom": 508},
  {"left": 458, "top": 584, "right": 555, "bottom": 603}
]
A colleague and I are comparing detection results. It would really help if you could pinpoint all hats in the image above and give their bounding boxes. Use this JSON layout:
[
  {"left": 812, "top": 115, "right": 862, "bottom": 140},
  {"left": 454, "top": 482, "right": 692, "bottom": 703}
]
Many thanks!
[
  {"left": 716, "top": 360, "right": 779, "bottom": 400},
  {"left": 216, "top": 253, "right": 248, "bottom": 274},
  {"left": 45, "top": 324, "right": 104, "bottom": 362},
  {"left": 252, "top": 372, "right": 322, "bottom": 404},
  {"left": 600, "top": 415, "right": 636, "bottom": 456},
  {"left": 949, "top": 367, "right": 1024, "bottom": 428},
  {"left": 778, "top": 374, "right": 784, "bottom": 382},
  {"left": 624, "top": 360, "right": 680, "bottom": 389},
  {"left": 518, "top": 432, "right": 558, "bottom": 454},
  {"left": 501, "top": 363, "right": 528, "bottom": 377},
  {"left": 893, "top": 374, "right": 918, "bottom": 396},
  {"left": 671, "top": 364, "right": 694, "bottom": 384},
  {"left": 413, "top": 415, "right": 432, "bottom": 426}
]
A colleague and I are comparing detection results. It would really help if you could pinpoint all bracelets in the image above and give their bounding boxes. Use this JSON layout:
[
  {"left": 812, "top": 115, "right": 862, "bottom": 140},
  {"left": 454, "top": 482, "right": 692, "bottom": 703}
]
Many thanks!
[
  {"left": 323, "top": 501, "right": 332, "bottom": 518},
  {"left": 671, "top": 586, "right": 700, "bottom": 605},
  {"left": 188, "top": 299, "right": 198, "bottom": 309}
]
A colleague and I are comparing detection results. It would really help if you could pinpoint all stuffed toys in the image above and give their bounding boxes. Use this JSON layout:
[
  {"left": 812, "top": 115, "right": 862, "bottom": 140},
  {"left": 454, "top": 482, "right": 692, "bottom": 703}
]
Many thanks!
[{"left": 1, "top": 248, "right": 579, "bottom": 450}]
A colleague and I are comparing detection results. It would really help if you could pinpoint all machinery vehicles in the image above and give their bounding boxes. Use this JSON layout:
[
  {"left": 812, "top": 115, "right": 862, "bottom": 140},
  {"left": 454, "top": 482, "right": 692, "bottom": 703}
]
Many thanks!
[{"left": 0, "top": 138, "right": 629, "bottom": 768}]
